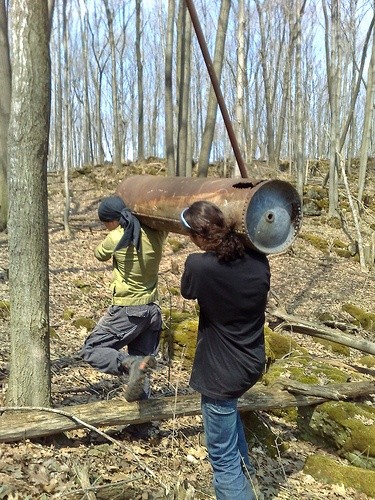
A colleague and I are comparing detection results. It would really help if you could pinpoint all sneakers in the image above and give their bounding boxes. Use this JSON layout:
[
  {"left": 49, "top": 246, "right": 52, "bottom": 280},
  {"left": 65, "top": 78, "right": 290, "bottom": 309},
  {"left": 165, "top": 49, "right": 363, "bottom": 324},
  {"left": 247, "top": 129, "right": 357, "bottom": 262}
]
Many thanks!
[
  {"left": 125, "top": 423, "right": 159, "bottom": 441},
  {"left": 123, "top": 355, "right": 156, "bottom": 402}
]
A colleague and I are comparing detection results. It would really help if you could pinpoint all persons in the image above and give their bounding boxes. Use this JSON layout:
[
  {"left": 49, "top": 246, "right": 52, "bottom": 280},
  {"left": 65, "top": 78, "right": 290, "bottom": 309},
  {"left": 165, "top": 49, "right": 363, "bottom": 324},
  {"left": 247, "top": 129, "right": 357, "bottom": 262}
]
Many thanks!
[
  {"left": 82, "top": 195, "right": 169, "bottom": 445},
  {"left": 180, "top": 200, "right": 271, "bottom": 500}
]
